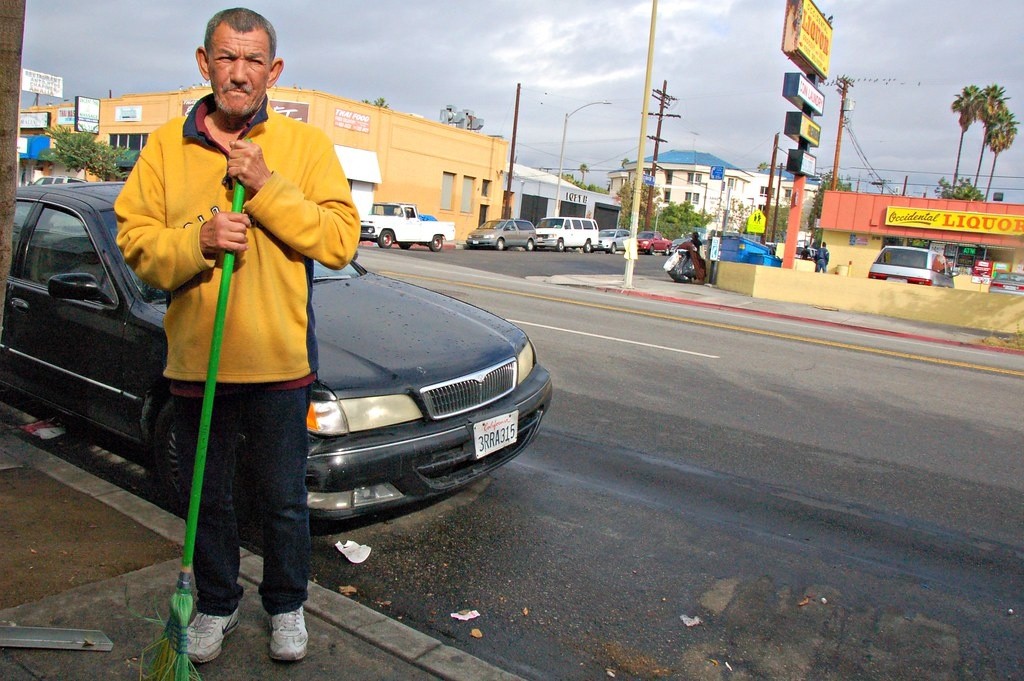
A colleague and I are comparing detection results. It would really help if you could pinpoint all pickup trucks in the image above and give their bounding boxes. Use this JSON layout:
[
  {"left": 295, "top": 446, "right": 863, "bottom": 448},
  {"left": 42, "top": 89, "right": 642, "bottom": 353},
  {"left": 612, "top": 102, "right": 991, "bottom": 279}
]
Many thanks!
[
  {"left": 637, "top": 231, "right": 673, "bottom": 256},
  {"left": 359, "top": 201, "right": 456, "bottom": 252}
]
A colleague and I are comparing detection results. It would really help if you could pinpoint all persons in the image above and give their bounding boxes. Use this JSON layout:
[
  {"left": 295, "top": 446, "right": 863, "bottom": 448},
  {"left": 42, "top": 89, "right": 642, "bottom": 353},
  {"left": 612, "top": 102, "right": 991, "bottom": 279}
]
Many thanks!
[
  {"left": 814, "top": 241, "right": 830, "bottom": 273},
  {"left": 690, "top": 232, "right": 702, "bottom": 255},
  {"left": 800, "top": 246, "right": 811, "bottom": 260},
  {"left": 112, "top": 8, "right": 361, "bottom": 666}
]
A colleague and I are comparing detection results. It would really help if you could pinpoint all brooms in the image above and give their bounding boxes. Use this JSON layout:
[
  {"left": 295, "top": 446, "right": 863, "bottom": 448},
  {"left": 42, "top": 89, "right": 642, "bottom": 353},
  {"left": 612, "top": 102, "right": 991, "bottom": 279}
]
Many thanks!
[{"left": 123, "top": 138, "right": 265, "bottom": 681}]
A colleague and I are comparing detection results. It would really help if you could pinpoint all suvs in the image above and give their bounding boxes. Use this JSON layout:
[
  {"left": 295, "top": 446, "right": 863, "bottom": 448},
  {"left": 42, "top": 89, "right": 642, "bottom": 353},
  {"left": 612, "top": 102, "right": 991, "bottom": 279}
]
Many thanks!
[{"left": 868, "top": 246, "right": 960, "bottom": 290}]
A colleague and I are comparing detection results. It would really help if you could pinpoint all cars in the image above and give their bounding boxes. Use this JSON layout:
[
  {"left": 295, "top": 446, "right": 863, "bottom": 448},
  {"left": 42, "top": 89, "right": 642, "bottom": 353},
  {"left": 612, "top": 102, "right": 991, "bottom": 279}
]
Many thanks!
[
  {"left": 592, "top": 228, "right": 630, "bottom": 254},
  {"left": 465, "top": 218, "right": 538, "bottom": 251},
  {"left": 794, "top": 246, "right": 817, "bottom": 260},
  {"left": 988, "top": 272, "right": 1024, "bottom": 296},
  {"left": 2, "top": 183, "right": 556, "bottom": 535},
  {"left": 670, "top": 237, "right": 688, "bottom": 253}
]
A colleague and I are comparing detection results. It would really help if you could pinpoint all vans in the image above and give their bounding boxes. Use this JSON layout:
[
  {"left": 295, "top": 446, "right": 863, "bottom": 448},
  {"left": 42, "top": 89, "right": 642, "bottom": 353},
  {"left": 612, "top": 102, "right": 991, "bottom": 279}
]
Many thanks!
[{"left": 534, "top": 217, "right": 599, "bottom": 253}]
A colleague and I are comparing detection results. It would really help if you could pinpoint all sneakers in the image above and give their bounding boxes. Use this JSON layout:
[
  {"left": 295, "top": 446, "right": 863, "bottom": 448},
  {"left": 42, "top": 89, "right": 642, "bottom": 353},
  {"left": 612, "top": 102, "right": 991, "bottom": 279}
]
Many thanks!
[
  {"left": 186, "top": 606, "right": 239, "bottom": 664},
  {"left": 268, "top": 604, "right": 309, "bottom": 660}
]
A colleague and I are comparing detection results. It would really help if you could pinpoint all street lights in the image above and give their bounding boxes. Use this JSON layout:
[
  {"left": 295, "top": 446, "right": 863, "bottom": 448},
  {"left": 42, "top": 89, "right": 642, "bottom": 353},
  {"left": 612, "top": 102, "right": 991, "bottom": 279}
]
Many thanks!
[{"left": 555, "top": 99, "right": 613, "bottom": 217}]
